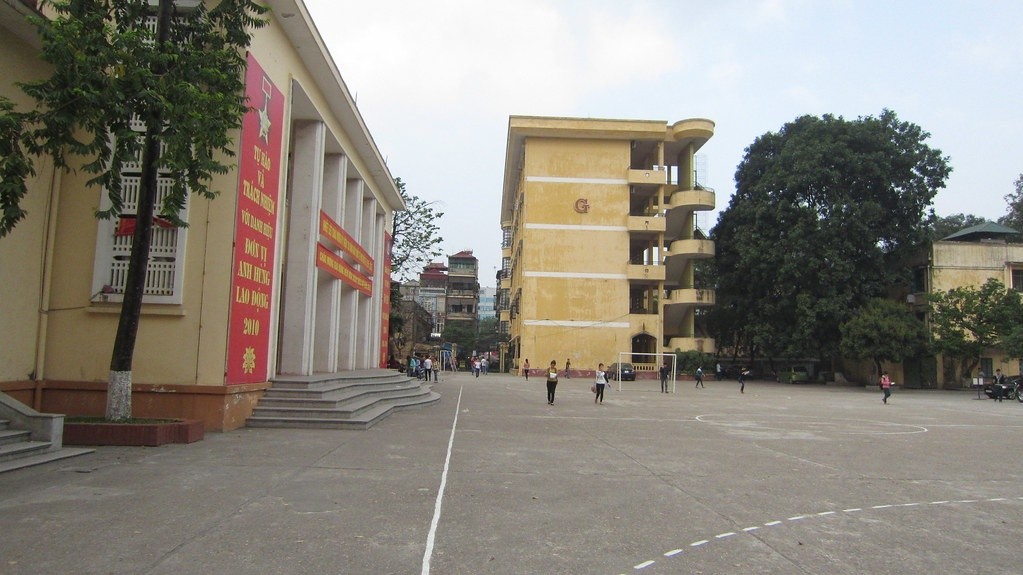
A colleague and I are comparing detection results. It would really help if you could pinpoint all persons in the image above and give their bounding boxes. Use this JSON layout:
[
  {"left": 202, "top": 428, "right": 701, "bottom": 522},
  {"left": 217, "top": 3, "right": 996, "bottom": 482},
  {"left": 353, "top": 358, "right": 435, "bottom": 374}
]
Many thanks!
[
  {"left": 423, "top": 355, "right": 432, "bottom": 381},
  {"left": 992, "top": 369, "right": 1004, "bottom": 403},
  {"left": 881, "top": 371, "right": 892, "bottom": 404},
  {"left": 660, "top": 362, "right": 670, "bottom": 393},
  {"left": 977, "top": 368, "right": 984, "bottom": 378},
  {"left": 388, "top": 354, "right": 425, "bottom": 380},
  {"left": 524, "top": 359, "right": 530, "bottom": 381},
  {"left": 716, "top": 361, "right": 723, "bottom": 381},
  {"left": 470, "top": 355, "right": 489, "bottom": 378},
  {"left": 564, "top": 358, "right": 571, "bottom": 379},
  {"left": 695, "top": 365, "right": 706, "bottom": 388},
  {"left": 594, "top": 363, "right": 610, "bottom": 405},
  {"left": 545, "top": 360, "right": 559, "bottom": 406},
  {"left": 738, "top": 366, "right": 749, "bottom": 393},
  {"left": 432, "top": 358, "right": 440, "bottom": 383}
]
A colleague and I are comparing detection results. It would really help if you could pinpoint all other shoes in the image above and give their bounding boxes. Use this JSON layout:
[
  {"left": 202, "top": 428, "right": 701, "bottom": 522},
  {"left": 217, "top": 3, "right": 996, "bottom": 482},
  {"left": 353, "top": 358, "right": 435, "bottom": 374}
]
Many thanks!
[
  {"left": 595, "top": 400, "right": 602, "bottom": 405},
  {"left": 548, "top": 400, "right": 553, "bottom": 405}
]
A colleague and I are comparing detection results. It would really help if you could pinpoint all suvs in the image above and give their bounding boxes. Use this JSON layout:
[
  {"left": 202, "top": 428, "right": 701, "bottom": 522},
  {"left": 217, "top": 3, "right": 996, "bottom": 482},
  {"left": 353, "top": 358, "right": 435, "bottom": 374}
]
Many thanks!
[{"left": 985, "top": 375, "right": 1023, "bottom": 400}]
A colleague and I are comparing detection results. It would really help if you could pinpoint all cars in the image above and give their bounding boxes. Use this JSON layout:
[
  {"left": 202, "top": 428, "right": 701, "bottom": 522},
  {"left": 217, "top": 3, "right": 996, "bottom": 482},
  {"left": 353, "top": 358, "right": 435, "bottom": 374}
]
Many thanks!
[
  {"left": 775, "top": 365, "right": 810, "bottom": 384},
  {"left": 607, "top": 363, "right": 636, "bottom": 381},
  {"left": 1014, "top": 377, "right": 1023, "bottom": 402}
]
